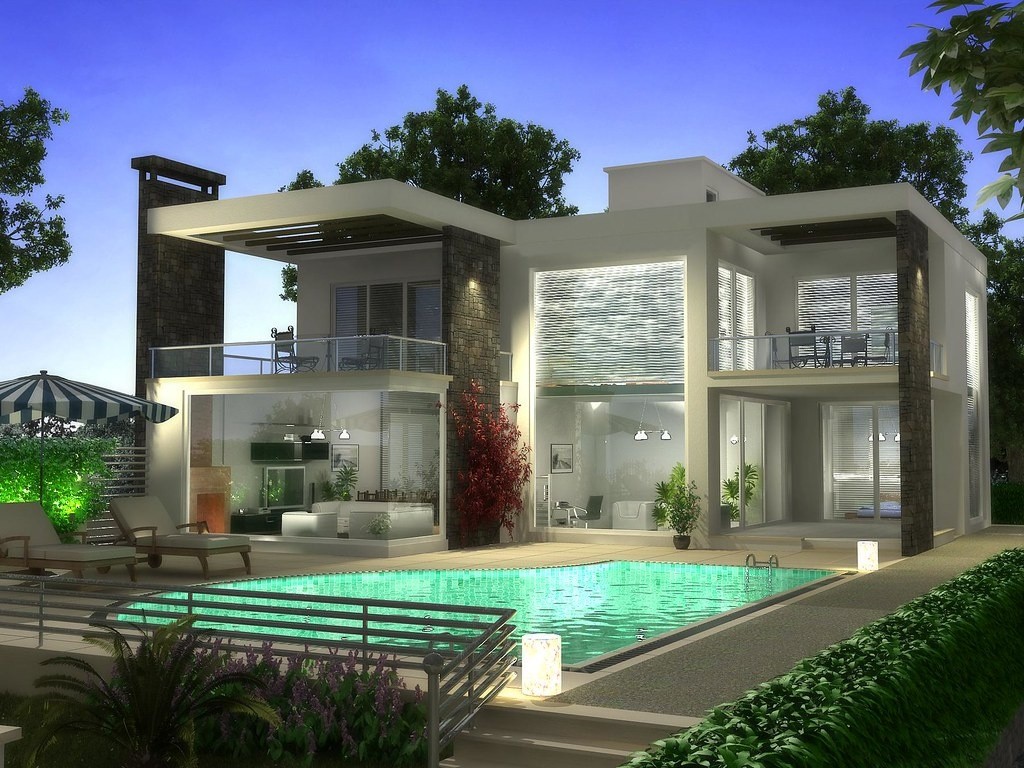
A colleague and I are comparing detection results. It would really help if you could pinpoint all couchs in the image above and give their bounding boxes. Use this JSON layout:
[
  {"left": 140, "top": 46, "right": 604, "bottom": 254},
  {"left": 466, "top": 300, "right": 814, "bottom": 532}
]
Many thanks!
[
  {"left": 611, "top": 500, "right": 659, "bottom": 532},
  {"left": 311, "top": 500, "right": 434, "bottom": 541},
  {"left": 281, "top": 511, "right": 339, "bottom": 539}
]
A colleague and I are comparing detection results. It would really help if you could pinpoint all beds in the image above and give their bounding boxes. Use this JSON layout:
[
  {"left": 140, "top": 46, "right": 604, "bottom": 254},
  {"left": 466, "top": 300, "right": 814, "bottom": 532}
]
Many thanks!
[{"left": 855, "top": 492, "right": 901, "bottom": 518}]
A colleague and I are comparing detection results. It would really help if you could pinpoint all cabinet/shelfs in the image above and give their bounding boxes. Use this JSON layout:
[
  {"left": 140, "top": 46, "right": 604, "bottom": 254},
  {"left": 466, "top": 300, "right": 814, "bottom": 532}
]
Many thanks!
[{"left": 229, "top": 510, "right": 291, "bottom": 534}]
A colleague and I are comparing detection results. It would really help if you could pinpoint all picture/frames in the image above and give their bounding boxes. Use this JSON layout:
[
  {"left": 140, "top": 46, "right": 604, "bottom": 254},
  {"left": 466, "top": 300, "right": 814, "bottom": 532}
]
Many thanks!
[
  {"left": 331, "top": 443, "right": 359, "bottom": 472},
  {"left": 551, "top": 443, "right": 573, "bottom": 474}
]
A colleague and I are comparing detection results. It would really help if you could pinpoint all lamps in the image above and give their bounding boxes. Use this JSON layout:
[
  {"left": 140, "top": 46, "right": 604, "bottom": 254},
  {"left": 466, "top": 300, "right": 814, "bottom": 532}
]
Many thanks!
[
  {"left": 310, "top": 393, "right": 349, "bottom": 440},
  {"left": 634, "top": 399, "right": 672, "bottom": 440}
]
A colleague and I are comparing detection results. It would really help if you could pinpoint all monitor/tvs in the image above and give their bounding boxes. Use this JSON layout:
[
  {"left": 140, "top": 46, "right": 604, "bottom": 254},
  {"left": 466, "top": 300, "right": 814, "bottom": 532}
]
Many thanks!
[{"left": 264, "top": 466, "right": 306, "bottom": 509}]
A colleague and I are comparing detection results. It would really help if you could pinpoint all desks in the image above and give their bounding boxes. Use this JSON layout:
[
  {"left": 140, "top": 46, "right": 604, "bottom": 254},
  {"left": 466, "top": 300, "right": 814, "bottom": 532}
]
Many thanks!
[{"left": 549, "top": 507, "right": 574, "bottom": 527}]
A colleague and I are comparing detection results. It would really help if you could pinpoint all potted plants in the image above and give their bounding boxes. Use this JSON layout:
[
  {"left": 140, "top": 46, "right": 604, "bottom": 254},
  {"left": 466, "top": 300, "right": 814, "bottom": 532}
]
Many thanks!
[{"left": 651, "top": 464, "right": 705, "bottom": 549}]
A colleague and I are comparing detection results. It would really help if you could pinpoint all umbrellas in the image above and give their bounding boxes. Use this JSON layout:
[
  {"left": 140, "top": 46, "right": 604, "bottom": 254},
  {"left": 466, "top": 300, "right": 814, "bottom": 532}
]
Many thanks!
[{"left": 0, "top": 370, "right": 180, "bottom": 508}]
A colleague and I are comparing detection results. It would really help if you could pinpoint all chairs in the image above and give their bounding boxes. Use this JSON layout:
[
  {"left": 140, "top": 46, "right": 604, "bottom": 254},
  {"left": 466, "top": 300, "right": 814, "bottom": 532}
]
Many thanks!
[
  {"left": 765, "top": 325, "right": 891, "bottom": 367},
  {"left": 569, "top": 495, "right": 603, "bottom": 529},
  {"left": 0, "top": 501, "right": 138, "bottom": 585},
  {"left": 270, "top": 326, "right": 319, "bottom": 373},
  {"left": 339, "top": 326, "right": 389, "bottom": 371},
  {"left": 109, "top": 494, "right": 252, "bottom": 579}
]
